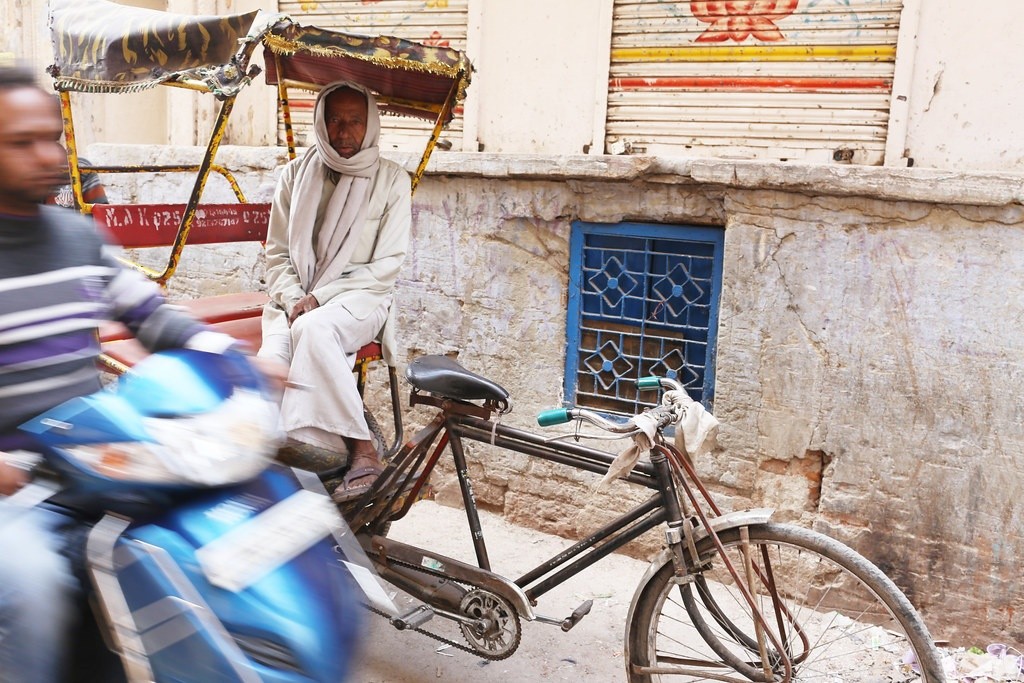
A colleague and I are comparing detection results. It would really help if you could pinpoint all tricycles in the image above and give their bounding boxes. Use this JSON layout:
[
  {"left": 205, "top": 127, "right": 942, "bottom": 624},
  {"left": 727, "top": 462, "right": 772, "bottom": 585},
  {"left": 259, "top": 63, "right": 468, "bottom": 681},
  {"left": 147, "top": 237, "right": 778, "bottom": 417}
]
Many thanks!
[{"left": 44, "top": 0, "right": 946, "bottom": 683}]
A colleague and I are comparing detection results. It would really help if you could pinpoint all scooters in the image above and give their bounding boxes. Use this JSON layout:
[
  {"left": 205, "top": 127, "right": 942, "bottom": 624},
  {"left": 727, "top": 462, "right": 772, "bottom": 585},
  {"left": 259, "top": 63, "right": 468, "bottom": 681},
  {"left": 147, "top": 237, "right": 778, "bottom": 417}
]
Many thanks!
[{"left": 18, "top": 346, "right": 370, "bottom": 683}]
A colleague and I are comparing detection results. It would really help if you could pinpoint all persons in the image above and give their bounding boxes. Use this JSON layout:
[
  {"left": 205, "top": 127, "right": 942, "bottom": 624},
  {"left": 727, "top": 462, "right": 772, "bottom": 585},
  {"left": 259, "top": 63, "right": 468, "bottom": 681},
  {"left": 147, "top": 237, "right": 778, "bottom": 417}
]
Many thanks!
[
  {"left": 254, "top": 79, "right": 414, "bottom": 504},
  {"left": 1, "top": 69, "right": 288, "bottom": 683}
]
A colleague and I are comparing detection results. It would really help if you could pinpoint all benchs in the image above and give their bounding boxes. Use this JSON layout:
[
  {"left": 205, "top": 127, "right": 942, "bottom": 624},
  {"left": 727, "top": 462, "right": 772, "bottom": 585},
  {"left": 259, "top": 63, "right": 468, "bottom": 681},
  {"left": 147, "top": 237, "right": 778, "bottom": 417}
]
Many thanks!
[{"left": 94, "top": 288, "right": 384, "bottom": 401}]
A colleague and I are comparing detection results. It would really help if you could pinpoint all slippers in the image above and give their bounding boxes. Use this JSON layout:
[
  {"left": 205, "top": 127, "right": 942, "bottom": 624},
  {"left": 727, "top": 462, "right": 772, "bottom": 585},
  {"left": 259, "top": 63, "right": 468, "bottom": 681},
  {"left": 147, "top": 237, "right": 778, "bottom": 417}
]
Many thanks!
[{"left": 331, "top": 466, "right": 386, "bottom": 502}]
